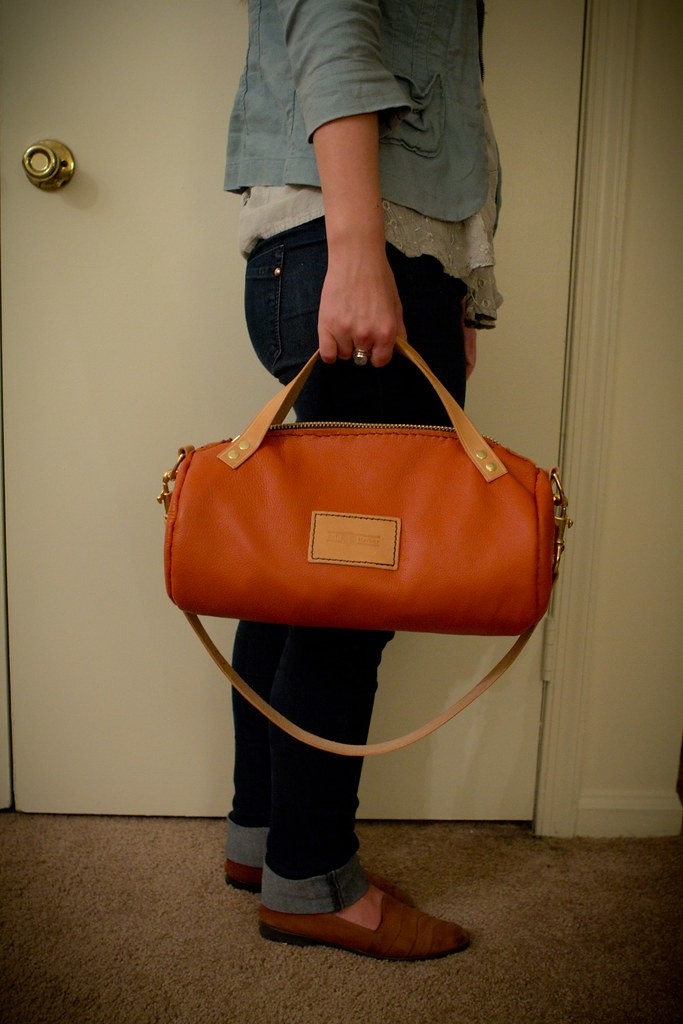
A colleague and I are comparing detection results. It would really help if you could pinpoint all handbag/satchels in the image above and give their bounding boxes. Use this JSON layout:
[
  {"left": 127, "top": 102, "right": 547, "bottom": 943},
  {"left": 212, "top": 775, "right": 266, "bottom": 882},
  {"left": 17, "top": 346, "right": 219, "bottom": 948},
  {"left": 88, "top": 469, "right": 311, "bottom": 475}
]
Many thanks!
[{"left": 161, "top": 337, "right": 575, "bottom": 756}]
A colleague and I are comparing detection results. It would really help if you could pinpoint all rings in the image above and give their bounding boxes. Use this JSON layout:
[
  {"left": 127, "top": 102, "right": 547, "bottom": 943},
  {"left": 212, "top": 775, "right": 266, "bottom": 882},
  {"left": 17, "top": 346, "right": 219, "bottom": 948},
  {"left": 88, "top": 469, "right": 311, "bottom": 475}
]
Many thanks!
[{"left": 353, "top": 348, "right": 368, "bottom": 367}]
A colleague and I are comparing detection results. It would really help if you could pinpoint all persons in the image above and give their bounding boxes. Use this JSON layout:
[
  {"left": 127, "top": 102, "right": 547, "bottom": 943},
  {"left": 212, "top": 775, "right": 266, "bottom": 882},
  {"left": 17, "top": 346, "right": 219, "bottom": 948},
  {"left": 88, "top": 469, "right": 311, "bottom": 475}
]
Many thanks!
[{"left": 221, "top": 0, "right": 502, "bottom": 962}]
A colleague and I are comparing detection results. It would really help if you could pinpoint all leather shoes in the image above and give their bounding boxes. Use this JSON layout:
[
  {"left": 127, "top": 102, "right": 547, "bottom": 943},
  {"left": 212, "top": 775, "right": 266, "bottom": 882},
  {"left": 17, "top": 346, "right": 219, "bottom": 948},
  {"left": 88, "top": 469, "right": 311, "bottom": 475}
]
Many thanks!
[{"left": 221, "top": 856, "right": 467, "bottom": 966}]
